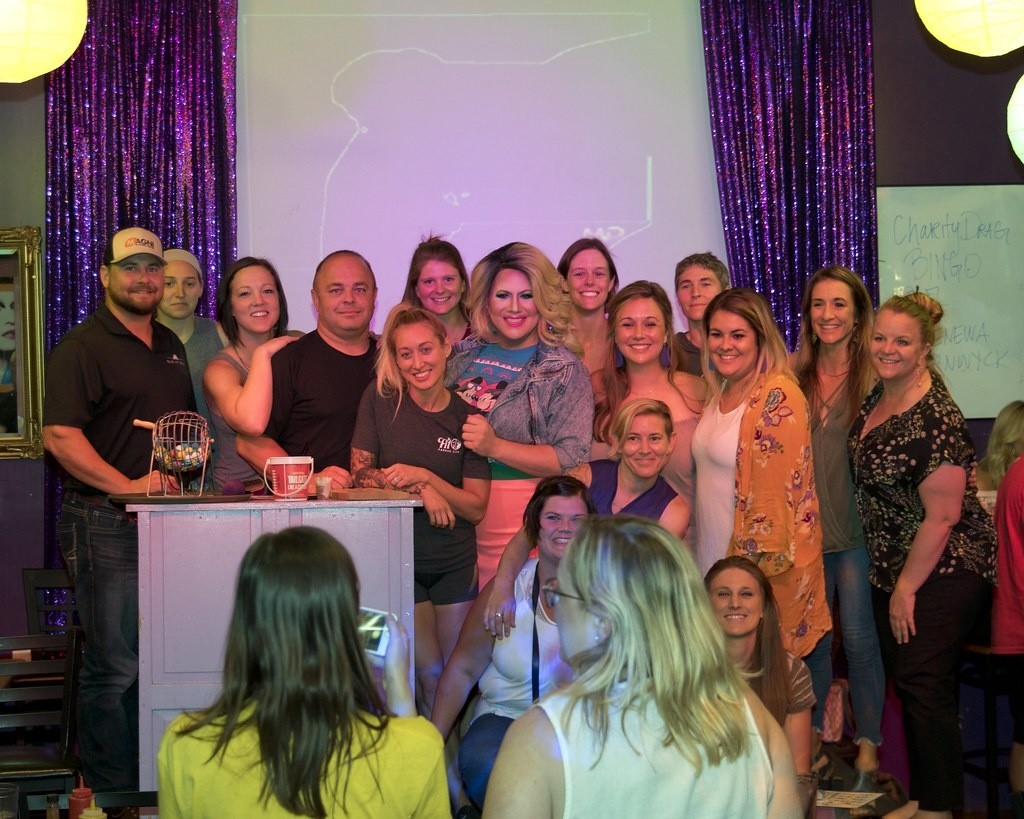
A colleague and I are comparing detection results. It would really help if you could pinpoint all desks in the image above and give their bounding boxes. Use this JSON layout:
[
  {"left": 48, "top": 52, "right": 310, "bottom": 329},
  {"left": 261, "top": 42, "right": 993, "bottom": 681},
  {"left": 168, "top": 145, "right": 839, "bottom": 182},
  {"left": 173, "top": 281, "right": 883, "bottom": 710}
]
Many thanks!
[{"left": 124, "top": 499, "right": 425, "bottom": 819}]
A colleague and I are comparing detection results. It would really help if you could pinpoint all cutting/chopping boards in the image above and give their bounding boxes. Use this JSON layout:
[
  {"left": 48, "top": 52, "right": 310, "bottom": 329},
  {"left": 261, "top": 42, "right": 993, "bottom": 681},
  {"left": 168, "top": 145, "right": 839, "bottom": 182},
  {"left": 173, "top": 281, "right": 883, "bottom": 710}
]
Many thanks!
[{"left": 331, "top": 488, "right": 410, "bottom": 500}]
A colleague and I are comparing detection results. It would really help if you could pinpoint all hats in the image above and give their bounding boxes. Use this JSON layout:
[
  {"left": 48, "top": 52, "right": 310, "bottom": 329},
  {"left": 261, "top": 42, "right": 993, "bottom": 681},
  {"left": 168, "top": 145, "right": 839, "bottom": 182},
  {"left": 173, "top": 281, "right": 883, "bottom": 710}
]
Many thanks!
[
  {"left": 104, "top": 227, "right": 168, "bottom": 266},
  {"left": 163, "top": 249, "right": 202, "bottom": 277}
]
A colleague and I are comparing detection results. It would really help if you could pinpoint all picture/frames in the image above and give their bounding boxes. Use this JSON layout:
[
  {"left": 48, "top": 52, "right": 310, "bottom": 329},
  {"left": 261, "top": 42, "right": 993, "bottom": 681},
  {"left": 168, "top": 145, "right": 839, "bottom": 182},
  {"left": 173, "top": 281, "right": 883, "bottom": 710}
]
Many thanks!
[{"left": 0, "top": 225, "right": 51, "bottom": 462}]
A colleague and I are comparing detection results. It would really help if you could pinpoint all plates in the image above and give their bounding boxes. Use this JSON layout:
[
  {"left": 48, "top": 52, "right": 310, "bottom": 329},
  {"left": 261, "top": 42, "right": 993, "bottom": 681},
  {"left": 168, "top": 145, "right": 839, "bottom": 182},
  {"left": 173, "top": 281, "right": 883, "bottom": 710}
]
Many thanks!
[{"left": 108, "top": 491, "right": 253, "bottom": 505}]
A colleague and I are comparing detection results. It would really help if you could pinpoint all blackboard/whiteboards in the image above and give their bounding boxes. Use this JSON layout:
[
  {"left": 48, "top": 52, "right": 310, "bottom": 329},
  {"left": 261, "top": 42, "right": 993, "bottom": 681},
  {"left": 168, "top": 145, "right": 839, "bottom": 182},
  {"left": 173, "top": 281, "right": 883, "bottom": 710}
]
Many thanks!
[{"left": 876, "top": 185, "right": 1024, "bottom": 419}]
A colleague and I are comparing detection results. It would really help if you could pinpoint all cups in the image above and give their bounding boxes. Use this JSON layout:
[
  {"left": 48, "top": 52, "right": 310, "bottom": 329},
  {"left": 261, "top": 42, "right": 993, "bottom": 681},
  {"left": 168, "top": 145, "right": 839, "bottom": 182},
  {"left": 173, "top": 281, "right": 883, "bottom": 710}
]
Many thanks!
[
  {"left": 314, "top": 473, "right": 333, "bottom": 500},
  {"left": 0, "top": 783, "right": 21, "bottom": 819}
]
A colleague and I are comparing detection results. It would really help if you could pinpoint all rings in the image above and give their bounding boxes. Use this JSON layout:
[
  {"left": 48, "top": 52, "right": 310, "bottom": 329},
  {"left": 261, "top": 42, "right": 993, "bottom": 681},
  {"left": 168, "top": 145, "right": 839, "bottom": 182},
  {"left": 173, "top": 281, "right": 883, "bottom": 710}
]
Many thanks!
[
  {"left": 394, "top": 477, "right": 399, "bottom": 483},
  {"left": 495, "top": 613, "right": 503, "bottom": 617}
]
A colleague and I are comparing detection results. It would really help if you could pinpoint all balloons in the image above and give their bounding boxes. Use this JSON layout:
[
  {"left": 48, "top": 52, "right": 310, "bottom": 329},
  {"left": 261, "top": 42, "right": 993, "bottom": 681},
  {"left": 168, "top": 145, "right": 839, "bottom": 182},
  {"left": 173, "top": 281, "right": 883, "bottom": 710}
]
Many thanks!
[
  {"left": 0, "top": 1, "right": 87, "bottom": 84},
  {"left": 915, "top": 0, "right": 1024, "bottom": 58},
  {"left": 1007, "top": 72, "right": 1024, "bottom": 168}
]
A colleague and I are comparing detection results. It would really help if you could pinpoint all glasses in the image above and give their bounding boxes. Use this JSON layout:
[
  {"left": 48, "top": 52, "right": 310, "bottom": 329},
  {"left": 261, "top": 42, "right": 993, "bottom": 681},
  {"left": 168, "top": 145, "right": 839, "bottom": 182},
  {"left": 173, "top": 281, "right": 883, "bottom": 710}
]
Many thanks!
[{"left": 542, "top": 576, "right": 583, "bottom": 609}]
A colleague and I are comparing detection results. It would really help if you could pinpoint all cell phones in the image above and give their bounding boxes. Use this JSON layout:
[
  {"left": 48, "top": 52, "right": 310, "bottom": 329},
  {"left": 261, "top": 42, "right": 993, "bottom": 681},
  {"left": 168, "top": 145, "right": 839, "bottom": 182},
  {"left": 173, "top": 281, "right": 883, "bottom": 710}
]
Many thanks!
[{"left": 358, "top": 607, "right": 398, "bottom": 656}]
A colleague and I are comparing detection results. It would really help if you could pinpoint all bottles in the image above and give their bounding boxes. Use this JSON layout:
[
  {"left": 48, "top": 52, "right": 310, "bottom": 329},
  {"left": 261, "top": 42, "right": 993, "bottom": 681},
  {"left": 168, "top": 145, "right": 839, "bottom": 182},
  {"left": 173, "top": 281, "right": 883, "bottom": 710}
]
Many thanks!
[
  {"left": 46, "top": 794, "right": 60, "bottom": 819},
  {"left": 78, "top": 798, "right": 108, "bottom": 819},
  {"left": 69, "top": 775, "right": 96, "bottom": 819}
]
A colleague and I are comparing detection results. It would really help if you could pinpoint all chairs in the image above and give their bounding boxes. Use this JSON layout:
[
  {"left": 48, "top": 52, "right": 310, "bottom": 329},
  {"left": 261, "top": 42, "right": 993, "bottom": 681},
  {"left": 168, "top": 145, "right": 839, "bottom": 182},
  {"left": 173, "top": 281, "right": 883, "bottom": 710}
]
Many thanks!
[{"left": 0, "top": 568, "right": 157, "bottom": 819}]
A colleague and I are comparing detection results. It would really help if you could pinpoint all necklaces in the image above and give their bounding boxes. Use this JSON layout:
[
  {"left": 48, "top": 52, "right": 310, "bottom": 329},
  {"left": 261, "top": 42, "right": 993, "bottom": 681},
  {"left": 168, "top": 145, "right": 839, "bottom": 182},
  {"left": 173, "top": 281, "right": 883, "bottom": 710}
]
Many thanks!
[
  {"left": 630, "top": 380, "right": 666, "bottom": 396},
  {"left": 234, "top": 346, "right": 250, "bottom": 370},
  {"left": 613, "top": 503, "right": 624, "bottom": 510}
]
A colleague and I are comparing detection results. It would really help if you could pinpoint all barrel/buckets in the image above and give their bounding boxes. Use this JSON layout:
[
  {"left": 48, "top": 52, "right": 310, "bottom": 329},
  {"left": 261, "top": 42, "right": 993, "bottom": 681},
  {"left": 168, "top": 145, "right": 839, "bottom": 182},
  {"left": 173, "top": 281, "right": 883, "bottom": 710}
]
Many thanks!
[{"left": 263, "top": 456, "right": 314, "bottom": 502}]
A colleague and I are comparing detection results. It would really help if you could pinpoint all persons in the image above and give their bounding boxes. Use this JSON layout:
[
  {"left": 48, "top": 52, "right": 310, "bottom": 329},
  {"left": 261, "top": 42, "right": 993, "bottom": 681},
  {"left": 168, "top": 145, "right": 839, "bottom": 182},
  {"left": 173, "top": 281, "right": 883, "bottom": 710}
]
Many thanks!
[
  {"left": 443, "top": 240, "right": 621, "bottom": 594},
  {"left": 787, "top": 264, "right": 1024, "bottom": 819},
  {"left": 375, "top": 230, "right": 473, "bottom": 362},
  {"left": 433, "top": 475, "right": 816, "bottom": 818},
  {"left": 41, "top": 227, "right": 204, "bottom": 794},
  {"left": 153, "top": 248, "right": 230, "bottom": 478},
  {"left": 664, "top": 249, "right": 832, "bottom": 660},
  {"left": 234, "top": 252, "right": 383, "bottom": 492},
  {"left": 349, "top": 304, "right": 492, "bottom": 819},
  {"left": 590, "top": 279, "right": 800, "bottom": 563},
  {"left": 483, "top": 399, "right": 692, "bottom": 641},
  {"left": 157, "top": 527, "right": 452, "bottom": 819},
  {"left": 201, "top": 257, "right": 306, "bottom": 492}
]
